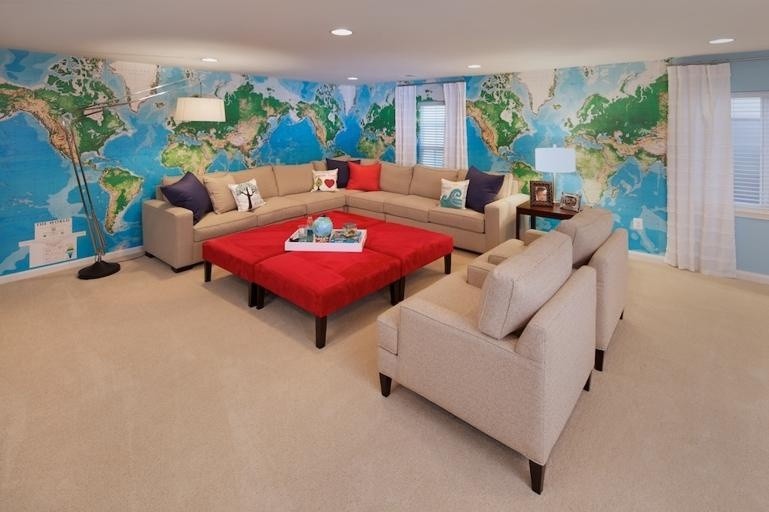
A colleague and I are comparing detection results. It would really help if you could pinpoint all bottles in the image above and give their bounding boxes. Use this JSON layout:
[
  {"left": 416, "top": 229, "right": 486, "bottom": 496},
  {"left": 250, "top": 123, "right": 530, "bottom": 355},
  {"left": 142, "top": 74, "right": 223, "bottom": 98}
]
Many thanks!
[{"left": 306, "top": 216, "right": 314, "bottom": 230}]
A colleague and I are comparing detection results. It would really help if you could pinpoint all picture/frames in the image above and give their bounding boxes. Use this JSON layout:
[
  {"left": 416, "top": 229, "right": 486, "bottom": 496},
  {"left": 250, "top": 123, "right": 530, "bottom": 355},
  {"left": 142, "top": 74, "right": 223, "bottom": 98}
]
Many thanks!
[
  {"left": 559, "top": 189, "right": 582, "bottom": 212},
  {"left": 529, "top": 179, "right": 554, "bottom": 208}
]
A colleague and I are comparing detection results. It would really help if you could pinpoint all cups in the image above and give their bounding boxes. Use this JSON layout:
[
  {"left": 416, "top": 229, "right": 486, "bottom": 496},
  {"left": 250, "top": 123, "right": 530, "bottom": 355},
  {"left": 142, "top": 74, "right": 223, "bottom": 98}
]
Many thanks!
[{"left": 298, "top": 225, "right": 307, "bottom": 241}]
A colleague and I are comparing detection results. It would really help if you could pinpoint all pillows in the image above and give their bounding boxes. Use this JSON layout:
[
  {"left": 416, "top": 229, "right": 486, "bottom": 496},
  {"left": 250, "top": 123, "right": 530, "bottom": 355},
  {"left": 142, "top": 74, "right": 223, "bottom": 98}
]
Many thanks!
[
  {"left": 409, "top": 164, "right": 467, "bottom": 200},
  {"left": 227, "top": 178, "right": 267, "bottom": 212},
  {"left": 437, "top": 177, "right": 468, "bottom": 210},
  {"left": 273, "top": 163, "right": 313, "bottom": 196},
  {"left": 456, "top": 168, "right": 513, "bottom": 202},
  {"left": 232, "top": 164, "right": 277, "bottom": 198},
  {"left": 311, "top": 168, "right": 339, "bottom": 193},
  {"left": 312, "top": 155, "right": 350, "bottom": 171},
  {"left": 558, "top": 205, "right": 614, "bottom": 264},
  {"left": 325, "top": 157, "right": 361, "bottom": 189},
  {"left": 464, "top": 165, "right": 505, "bottom": 214},
  {"left": 202, "top": 172, "right": 237, "bottom": 214},
  {"left": 380, "top": 161, "right": 414, "bottom": 194},
  {"left": 345, "top": 161, "right": 383, "bottom": 192},
  {"left": 475, "top": 228, "right": 576, "bottom": 340},
  {"left": 159, "top": 170, "right": 213, "bottom": 226}
]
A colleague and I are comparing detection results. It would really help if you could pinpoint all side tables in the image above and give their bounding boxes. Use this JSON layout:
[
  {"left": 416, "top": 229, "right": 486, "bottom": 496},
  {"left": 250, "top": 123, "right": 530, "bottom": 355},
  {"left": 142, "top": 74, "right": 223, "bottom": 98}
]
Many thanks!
[{"left": 515, "top": 199, "right": 581, "bottom": 241}]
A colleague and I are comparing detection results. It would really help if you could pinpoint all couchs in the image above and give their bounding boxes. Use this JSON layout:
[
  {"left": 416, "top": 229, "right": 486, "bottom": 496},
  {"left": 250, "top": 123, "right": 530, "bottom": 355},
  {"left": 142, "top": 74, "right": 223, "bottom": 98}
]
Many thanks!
[
  {"left": 374, "top": 260, "right": 598, "bottom": 494},
  {"left": 464, "top": 227, "right": 629, "bottom": 373},
  {"left": 141, "top": 153, "right": 344, "bottom": 274},
  {"left": 347, "top": 157, "right": 533, "bottom": 254}
]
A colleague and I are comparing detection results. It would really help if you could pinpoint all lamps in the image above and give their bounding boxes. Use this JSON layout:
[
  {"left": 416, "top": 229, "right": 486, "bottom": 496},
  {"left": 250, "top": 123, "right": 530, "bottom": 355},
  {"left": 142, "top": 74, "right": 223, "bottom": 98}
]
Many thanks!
[
  {"left": 57, "top": 72, "right": 227, "bottom": 279},
  {"left": 535, "top": 143, "right": 578, "bottom": 202}
]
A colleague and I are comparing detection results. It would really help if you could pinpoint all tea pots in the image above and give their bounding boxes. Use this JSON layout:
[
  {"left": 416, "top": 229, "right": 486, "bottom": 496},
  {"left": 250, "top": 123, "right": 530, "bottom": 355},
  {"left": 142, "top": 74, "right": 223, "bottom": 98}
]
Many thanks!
[{"left": 341, "top": 221, "right": 358, "bottom": 239}]
